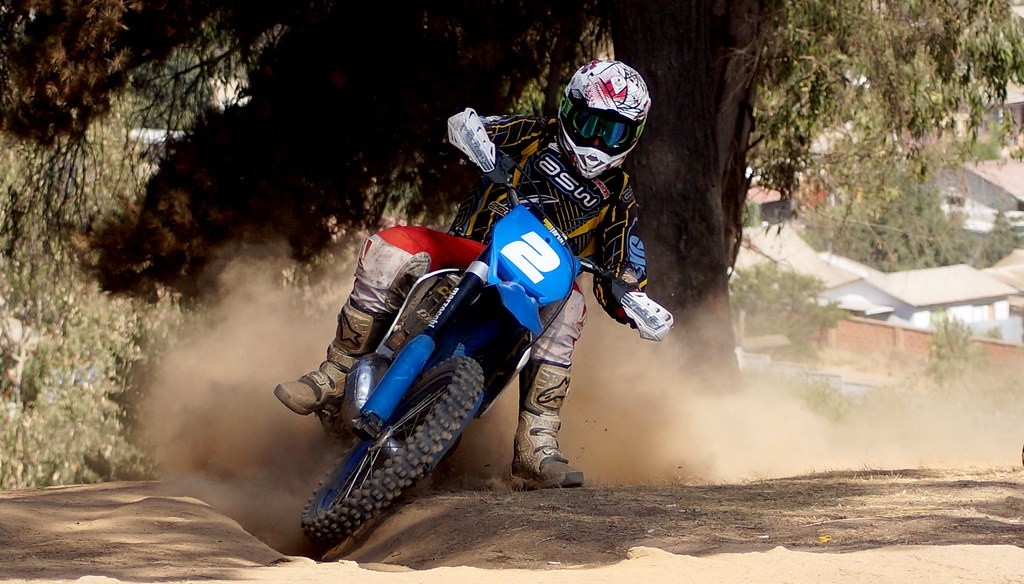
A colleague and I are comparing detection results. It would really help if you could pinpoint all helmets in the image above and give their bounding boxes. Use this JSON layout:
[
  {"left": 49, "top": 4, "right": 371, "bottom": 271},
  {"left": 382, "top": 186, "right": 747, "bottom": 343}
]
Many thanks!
[{"left": 558, "top": 60, "right": 651, "bottom": 178}]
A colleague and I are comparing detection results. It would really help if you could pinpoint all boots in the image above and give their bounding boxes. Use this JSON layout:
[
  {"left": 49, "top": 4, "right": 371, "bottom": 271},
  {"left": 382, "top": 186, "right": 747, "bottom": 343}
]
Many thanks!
[
  {"left": 510, "top": 358, "right": 583, "bottom": 490},
  {"left": 274, "top": 297, "right": 396, "bottom": 415}
]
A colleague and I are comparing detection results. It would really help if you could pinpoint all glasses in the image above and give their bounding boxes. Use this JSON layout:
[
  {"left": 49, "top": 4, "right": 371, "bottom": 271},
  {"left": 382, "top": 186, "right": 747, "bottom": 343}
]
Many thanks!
[{"left": 569, "top": 103, "right": 642, "bottom": 149}]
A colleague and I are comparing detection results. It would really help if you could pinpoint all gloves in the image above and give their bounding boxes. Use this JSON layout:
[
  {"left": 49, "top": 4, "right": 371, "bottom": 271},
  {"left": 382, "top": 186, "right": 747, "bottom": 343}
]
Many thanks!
[{"left": 615, "top": 288, "right": 642, "bottom": 329}]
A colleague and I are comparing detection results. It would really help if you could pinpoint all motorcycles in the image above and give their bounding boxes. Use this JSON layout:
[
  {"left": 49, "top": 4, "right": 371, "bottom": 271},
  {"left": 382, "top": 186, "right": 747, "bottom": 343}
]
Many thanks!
[{"left": 301, "top": 108, "right": 675, "bottom": 547}]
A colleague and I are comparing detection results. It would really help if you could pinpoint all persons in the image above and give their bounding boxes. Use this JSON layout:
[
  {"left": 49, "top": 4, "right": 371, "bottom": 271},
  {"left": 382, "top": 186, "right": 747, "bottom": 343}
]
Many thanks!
[{"left": 272, "top": 59, "right": 653, "bottom": 486}]
